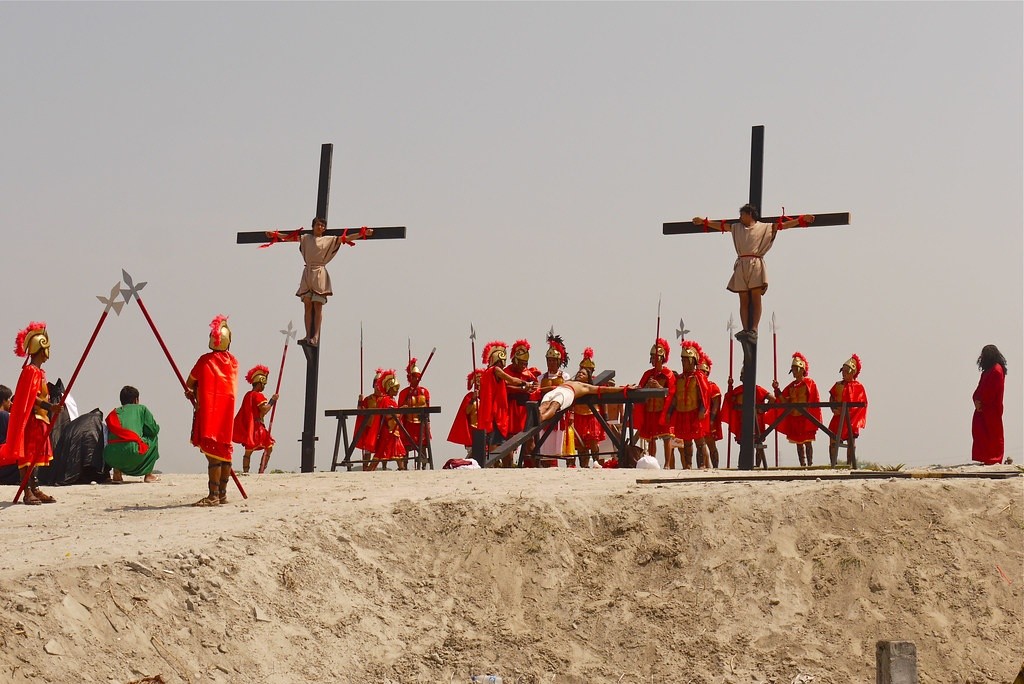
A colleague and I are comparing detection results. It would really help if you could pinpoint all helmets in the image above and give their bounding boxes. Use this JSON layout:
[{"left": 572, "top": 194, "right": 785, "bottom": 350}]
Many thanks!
[
  {"left": 209, "top": 314, "right": 231, "bottom": 351},
  {"left": 579, "top": 347, "right": 595, "bottom": 373},
  {"left": 481, "top": 342, "right": 507, "bottom": 367},
  {"left": 697, "top": 353, "right": 712, "bottom": 376},
  {"left": 839, "top": 354, "right": 861, "bottom": 381},
  {"left": 650, "top": 338, "right": 670, "bottom": 365},
  {"left": 13, "top": 321, "right": 50, "bottom": 359},
  {"left": 467, "top": 368, "right": 485, "bottom": 391},
  {"left": 739, "top": 366, "right": 744, "bottom": 381},
  {"left": 372, "top": 368, "right": 384, "bottom": 388},
  {"left": 510, "top": 340, "right": 530, "bottom": 364},
  {"left": 406, "top": 358, "right": 420, "bottom": 383},
  {"left": 546, "top": 333, "right": 570, "bottom": 368},
  {"left": 681, "top": 341, "right": 704, "bottom": 365},
  {"left": 789, "top": 351, "right": 809, "bottom": 378},
  {"left": 246, "top": 365, "right": 270, "bottom": 389},
  {"left": 378, "top": 369, "right": 399, "bottom": 394}
]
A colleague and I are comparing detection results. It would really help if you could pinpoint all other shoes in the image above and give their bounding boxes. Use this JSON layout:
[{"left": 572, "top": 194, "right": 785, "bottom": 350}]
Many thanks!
[
  {"left": 220, "top": 495, "right": 228, "bottom": 504},
  {"left": 746, "top": 331, "right": 756, "bottom": 339},
  {"left": 735, "top": 331, "right": 746, "bottom": 336},
  {"left": 23, "top": 495, "right": 41, "bottom": 505},
  {"left": 35, "top": 491, "right": 56, "bottom": 502},
  {"left": 193, "top": 498, "right": 220, "bottom": 506}
]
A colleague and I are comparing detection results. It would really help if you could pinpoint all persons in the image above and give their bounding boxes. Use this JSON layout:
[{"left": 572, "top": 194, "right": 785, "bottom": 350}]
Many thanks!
[
  {"left": 353, "top": 358, "right": 431, "bottom": 471},
  {"left": 720, "top": 365, "right": 776, "bottom": 447},
  {"left": 184, "top": 314, "right": 240, "bottom": 506},
  {"left": 232, "top": 365, "right": 279, "bottom": 474},
  {"left": 692, "top": 204, "right": 815, "bottom": 339},
  {"left": 448, "top": 334, "right": 625, "bottom": 469},
  {"left": 103, "top": 385, "right": 161, "bottom": 483},
  {"left": 826, "top": 353, "right": 868, "bottom": 467},
  {"left": 628, "top": 338, "right": 721, "bottom": 470},
  {"left": 5, "top": 320, "right": 64, "bottom": 505},
  {"left": 266, "top": 217, "right": 373, "bottom": 345},
  {"left": 972, "top": 344, "right": 1007, "bottom": 465},
  {"left": 772, "top": 353, "right": 823, "bottom": 467},
  {"left": 0, "top": 379, "right": 108, "bottom": 487}
]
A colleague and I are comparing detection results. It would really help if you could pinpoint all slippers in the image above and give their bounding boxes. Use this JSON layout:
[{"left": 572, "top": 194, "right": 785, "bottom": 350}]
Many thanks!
[
  {"left": 145, "top": 477, "right": 161, "bottom": 483},
  {"left": 112, "top": 476, "right": 123, "bottom": 483}
]
